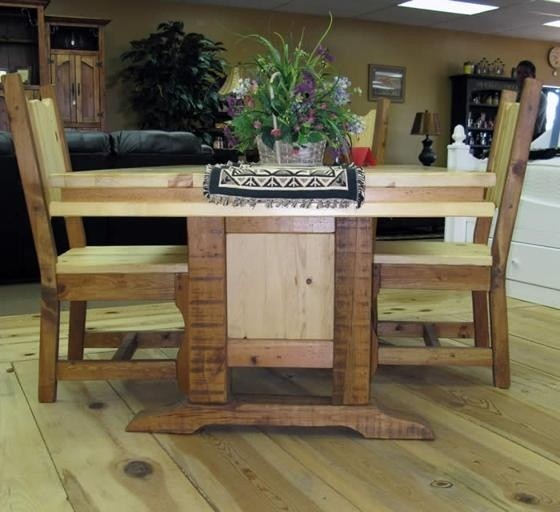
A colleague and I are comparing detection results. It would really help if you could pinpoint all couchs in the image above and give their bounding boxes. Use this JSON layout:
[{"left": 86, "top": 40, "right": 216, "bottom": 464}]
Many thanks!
[{"left": 0, "top": 127, "right": 214, "bottom": 284}]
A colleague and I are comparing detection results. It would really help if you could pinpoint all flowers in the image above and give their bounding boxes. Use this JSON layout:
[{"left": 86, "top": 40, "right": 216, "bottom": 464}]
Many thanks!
[{"left": 225, "top": 12, "right": 363, "bottom": 164}]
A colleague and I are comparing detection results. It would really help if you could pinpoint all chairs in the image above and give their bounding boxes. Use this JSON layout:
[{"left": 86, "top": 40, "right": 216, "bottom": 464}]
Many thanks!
[
  {"left": 374, "top": 78, "right": 542, "bottom": 389},
  {"left": 2, "top": 74, "right": 189, "bottom": 404},
  {"left": 324, "top": 99, "right": 390, "bottom": 165}
]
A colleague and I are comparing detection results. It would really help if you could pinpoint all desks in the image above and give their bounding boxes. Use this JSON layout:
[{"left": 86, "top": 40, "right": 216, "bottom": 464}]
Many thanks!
[{"left": 51, "top": 164, "right": 495, "bottom": 440}]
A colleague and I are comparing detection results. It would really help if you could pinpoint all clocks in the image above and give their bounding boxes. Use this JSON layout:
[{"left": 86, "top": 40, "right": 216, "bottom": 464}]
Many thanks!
[{"left": 549, "top": 46, "right": 560, "bottom": 69}]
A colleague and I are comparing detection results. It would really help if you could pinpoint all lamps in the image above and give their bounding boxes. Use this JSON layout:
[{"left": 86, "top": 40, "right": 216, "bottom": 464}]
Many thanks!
[{"left": 410, "top": 110, "right": 442, "bottom": 166}]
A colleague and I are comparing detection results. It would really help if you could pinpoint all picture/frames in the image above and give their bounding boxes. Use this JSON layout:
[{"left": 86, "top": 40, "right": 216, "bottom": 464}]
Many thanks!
[{"left": 368, "top": 64, "right": 406, "bottom": 103}]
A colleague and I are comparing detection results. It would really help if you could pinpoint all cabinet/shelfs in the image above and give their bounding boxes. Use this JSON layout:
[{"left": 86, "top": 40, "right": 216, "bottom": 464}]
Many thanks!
[
  {"left": 45, "top": 15, "right": 111, "bottom": 132},
  {"left": 450, "top": 74, "right": 523, "bottom": 159}
]
